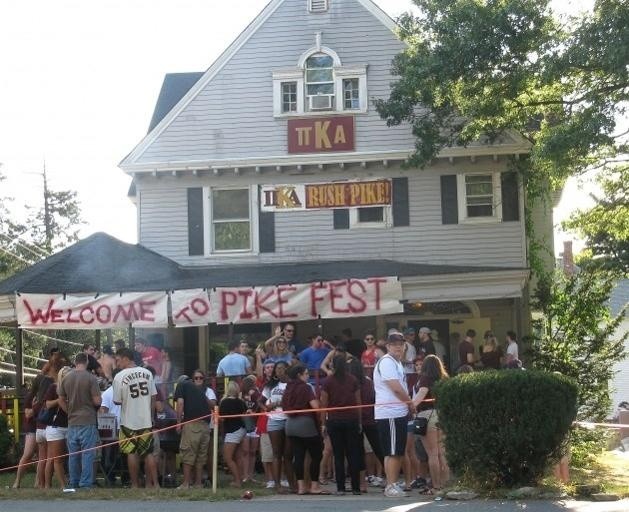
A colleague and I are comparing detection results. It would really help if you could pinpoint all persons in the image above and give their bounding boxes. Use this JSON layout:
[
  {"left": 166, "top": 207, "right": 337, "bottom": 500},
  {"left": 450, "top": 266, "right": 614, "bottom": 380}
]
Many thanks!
[
  {"left": 556, "top": 454, "right": 570, "bottom": 480},
  {"left": 13, "top": 322, "right": 451, "bottom": 497},
  {"left": 457, "top": 329, "right": 522, "bottom": 373}
]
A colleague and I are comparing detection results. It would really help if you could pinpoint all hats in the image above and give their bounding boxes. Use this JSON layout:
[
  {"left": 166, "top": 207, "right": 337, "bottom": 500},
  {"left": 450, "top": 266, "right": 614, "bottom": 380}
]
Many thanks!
[
  {"left": 430, "top": 329, "right": 439, "bottom": 341},
  {"left": 383, "top": 333, "right": 406, "bottom": 345},
  {"left": 419, "top": 327, "right": 432, "bottom": 334},
  {"left": 406, "top": 327, "right": 416, "bottom": 334}
]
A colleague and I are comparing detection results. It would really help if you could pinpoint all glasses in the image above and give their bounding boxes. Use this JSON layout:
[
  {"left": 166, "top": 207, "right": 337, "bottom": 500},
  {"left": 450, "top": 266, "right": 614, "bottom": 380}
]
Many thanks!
[{"left": 195, "top": 377, "right": 203, "bottom": 381}]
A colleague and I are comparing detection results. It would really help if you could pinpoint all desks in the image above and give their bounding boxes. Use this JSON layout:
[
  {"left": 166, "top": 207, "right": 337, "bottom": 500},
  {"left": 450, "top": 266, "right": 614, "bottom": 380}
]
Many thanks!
[{"left": 98, "top": 439, "right": 180, "bottom": 488}]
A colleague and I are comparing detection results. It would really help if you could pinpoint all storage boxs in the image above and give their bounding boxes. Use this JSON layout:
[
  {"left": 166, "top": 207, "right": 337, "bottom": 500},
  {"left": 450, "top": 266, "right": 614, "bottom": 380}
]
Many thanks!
[{"left": 97, "top": 413, "right": 117, "bottom": 440}]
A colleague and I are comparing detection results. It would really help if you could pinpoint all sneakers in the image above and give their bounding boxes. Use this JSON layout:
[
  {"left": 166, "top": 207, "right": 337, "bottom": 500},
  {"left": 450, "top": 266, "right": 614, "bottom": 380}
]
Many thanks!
[{"left": 266, "top": 474, "right": 444, "bottom": 498}]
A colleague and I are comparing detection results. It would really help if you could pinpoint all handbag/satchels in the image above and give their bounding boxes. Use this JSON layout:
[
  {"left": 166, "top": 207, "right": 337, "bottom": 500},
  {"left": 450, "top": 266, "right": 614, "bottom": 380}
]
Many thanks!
[{"left": 413, "top": 417, "right": 428, "bottom": 436}]
